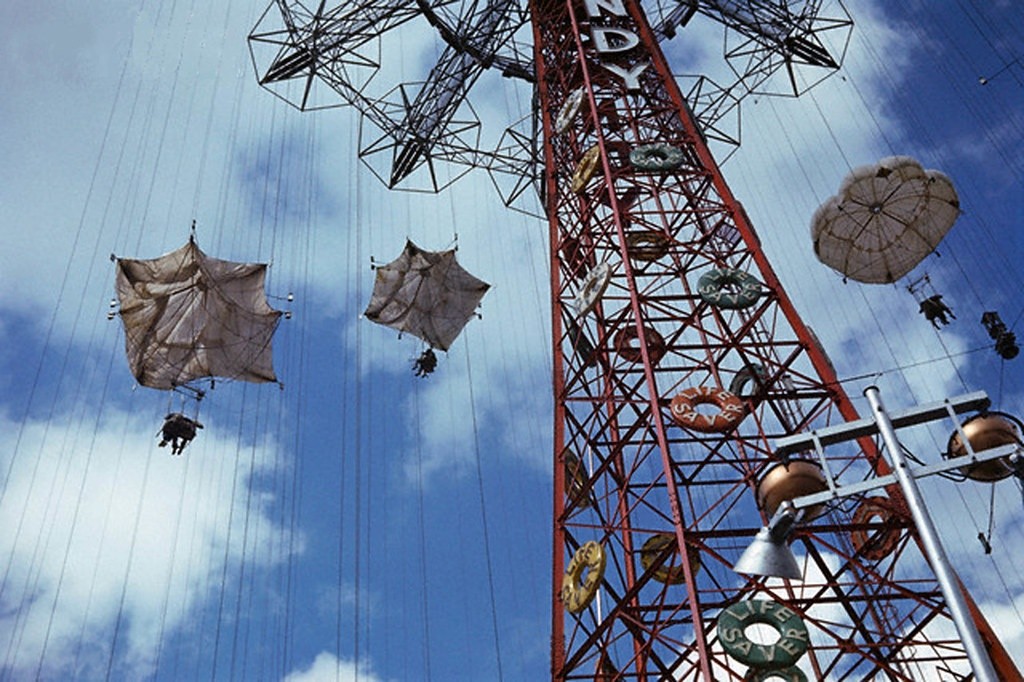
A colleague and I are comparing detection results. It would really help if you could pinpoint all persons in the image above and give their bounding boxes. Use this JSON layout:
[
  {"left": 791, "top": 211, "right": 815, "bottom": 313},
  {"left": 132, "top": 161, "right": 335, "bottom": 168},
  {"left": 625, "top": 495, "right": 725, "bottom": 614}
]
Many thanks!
[
  {"left": 411, "top": 348, "right": 437, "bottom": 376},
  {"left": 171, "top": 413, "right": 204, "bottom": 454},
  {"left": 421, "top": 357, "right": 437, "bottom": 378},
  {"left": 930, "top": 295, "right": 956, "bottom": 325},
  {"left": 158, "top": 412, "right": 183, "bottom": 447},
  {"left": 919, "top": 300, "right": 950, "bottom": 330}
]
